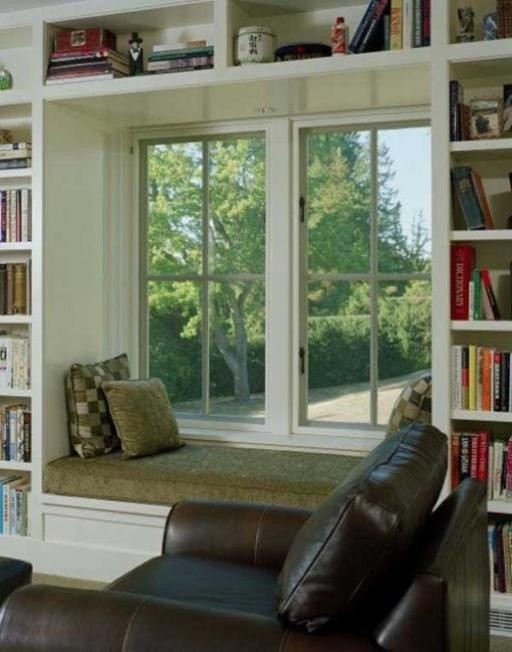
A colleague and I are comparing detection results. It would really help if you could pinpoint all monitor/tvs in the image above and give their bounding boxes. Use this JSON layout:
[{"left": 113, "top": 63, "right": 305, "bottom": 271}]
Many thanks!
[
  {"left": 450, "top": 80, "right": 511, "bottom": 593},
  {"left": 346, "top": 1, "right": 431, "bottom": 54},
  {"left": 0, "top": 142, "right": 32, "bottom": 534},
  {"left": 46, "top": 38, "right": 214, "bottom": 85}
]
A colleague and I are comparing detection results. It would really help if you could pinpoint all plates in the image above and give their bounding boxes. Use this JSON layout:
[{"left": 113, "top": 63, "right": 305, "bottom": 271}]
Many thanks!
[
  {"left": 1, "top": 2, "right": 41, "bottom": 565},
  {"left": 434, "top": 0, "right": 512, "bottom": 642}
]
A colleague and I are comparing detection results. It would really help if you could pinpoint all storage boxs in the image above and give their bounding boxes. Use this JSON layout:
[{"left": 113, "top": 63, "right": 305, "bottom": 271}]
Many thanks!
[{"left": 38, "top": 426, "right": 365, "bottom": 581}]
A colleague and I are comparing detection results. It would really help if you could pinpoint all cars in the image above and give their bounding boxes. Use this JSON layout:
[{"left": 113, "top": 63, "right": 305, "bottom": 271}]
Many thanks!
[{"left": 232, "top": 24, "right": 277, "bottom": 65}]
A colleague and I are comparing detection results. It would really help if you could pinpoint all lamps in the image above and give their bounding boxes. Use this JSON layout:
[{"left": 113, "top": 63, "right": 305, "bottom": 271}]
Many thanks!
[
  {"left": 1, "top": 553, "right": 34, "bottom": 608},
  {"left": 2, "top": 431, "right": 495, "bottom": 649}
]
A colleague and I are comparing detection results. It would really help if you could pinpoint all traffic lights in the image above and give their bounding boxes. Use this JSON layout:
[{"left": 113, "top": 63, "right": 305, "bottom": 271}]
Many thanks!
[
  {"left": 386, "top": 376, "right": 436, "bottom": 451},
  {"left": 66, "top": 351, "right": 134, "bottom": 462},
  {"left": 100, "top": 372, "right": 188, "bottom": 462}
]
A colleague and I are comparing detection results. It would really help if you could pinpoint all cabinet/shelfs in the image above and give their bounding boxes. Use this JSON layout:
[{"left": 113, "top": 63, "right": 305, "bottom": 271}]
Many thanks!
[
  {"left": 0, "top": 61, "right": 13, "bottom": 90},
  {"left": 331, "top": 14, "right": 349, "bottom": 54}
]
